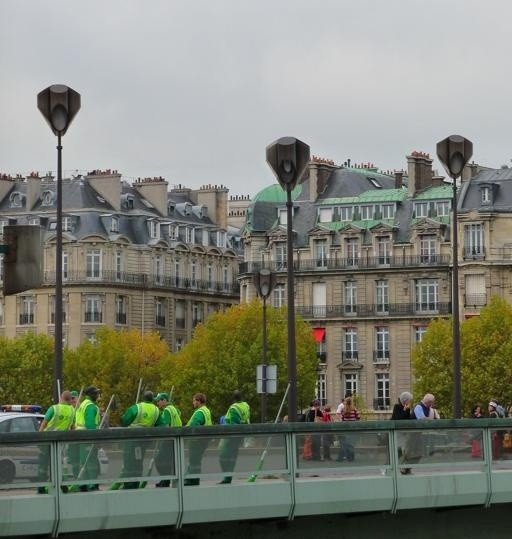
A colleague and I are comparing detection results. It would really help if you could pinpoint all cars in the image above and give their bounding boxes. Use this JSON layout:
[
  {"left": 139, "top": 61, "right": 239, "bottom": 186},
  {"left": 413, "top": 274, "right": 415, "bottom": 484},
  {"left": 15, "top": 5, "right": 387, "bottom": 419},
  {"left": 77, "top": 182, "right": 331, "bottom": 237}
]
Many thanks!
[{"left": 0, "top": 401, "right": 111, "bottom": 484}]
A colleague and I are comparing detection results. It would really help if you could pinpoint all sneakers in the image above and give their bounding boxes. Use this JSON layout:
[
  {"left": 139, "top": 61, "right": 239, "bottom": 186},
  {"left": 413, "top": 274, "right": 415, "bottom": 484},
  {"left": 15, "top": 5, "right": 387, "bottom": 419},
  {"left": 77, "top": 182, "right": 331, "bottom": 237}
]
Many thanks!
[
  {"left": 303, "top": 455, "right": 355, "bottom": 463},
  {"left": 489, "top": 401, "right": 497, "bottom": 408}
]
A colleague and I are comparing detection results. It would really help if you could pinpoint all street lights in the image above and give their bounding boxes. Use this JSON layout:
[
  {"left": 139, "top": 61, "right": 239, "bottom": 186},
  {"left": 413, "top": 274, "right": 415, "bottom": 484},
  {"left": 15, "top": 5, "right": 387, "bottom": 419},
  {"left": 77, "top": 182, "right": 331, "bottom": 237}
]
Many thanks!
[
  {"left": 255, "top": 266, "right": 276, "bottom": 424},
  {"left": 35, "top": 79, "right": 87, "bottom": 398},
  {"left": 432, "top": 134, "right": 478, "bottom": 418},
  {"left": 264, "top": 132, "right": 311, "bottom": 479}
]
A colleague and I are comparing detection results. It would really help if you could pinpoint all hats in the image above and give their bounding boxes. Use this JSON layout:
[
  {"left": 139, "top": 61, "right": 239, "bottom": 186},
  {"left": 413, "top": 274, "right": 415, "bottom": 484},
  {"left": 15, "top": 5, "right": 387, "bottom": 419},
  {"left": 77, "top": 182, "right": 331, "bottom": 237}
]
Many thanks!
[
  {"left": 85, "top": 385, "right": 100, "bottom": 395},
  {"left": 153, "top": 392, "right": 169, "bottom": 402},
  {"left": 70, "top": 390, "right": 79, "bottom": 398}
]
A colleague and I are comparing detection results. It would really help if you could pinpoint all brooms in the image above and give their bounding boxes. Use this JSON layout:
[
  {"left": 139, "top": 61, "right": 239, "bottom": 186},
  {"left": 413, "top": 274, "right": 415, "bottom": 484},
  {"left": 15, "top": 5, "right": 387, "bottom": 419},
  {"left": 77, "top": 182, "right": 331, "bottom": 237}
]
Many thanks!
[
  {"left": 57, "top": 379, "right": 69, "bottom": 494},
  {"left": 107, "top": 377, "right": 143, "bottom": 490},
  {"left": 138, "top": 384, "right": 175, "bottom": 487},
  {"left": 248, "top": 382, "right": 290, "bottom": 482},
  {"left": 44, "top": 383, "right": 86, "bottom": 493},
  {"left": 68, "top": 394, "right": 116, "bottom": 492}
]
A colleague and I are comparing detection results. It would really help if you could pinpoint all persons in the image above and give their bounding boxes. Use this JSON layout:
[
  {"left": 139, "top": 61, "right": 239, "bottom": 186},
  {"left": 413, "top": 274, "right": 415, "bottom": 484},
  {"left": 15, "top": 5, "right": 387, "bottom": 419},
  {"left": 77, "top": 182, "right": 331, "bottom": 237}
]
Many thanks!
[
  {"left": 120, "top": 390, "right": 161, "bottom": 488},
  {"left": 153, "top": 392, "right": 183, "bottom": 487},
  {"left": 298, "top": 396, "right": 361, "bottom": 462},
  {"left": 66, "top": 390, "right": 81, "bottom": 479},
  {"left": 399, "top": 394, "right": 441, "bottom": 474},
  {"left": 216, "top": 389, "right": 250, "bottom": 484},
  {"left": 73, "top": 386, "right": 101, "bottom": 492},
  {"left": 380, "top": 390, "right": 415, "bottom": 476},
  {"left": 183, "top": 393, "right": 213, "bottom": 486},
  {"left": 37, "top": 391, "right": 75, "bottom": 493},
  {"left": 470, "top": 401, "right": 506, "bottom": 460}
]
